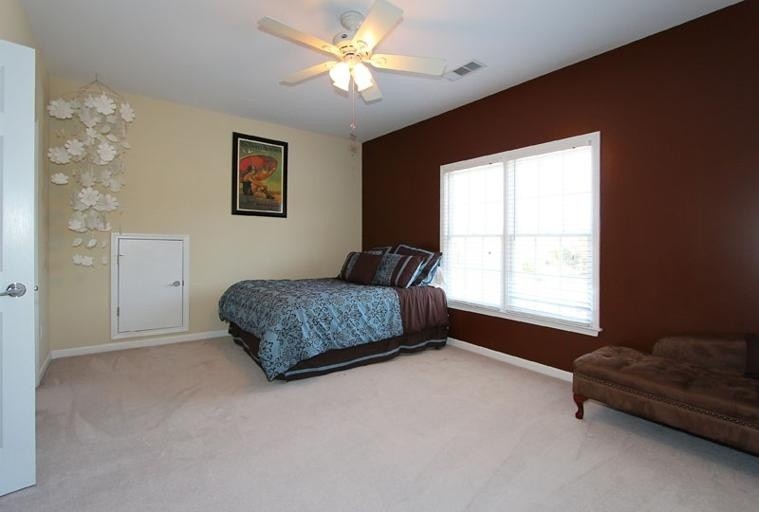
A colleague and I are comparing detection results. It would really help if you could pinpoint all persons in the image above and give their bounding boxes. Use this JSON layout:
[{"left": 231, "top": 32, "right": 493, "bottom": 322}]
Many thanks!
[{"left": 243, "top": 165, "right": 275, "bottom": 200}]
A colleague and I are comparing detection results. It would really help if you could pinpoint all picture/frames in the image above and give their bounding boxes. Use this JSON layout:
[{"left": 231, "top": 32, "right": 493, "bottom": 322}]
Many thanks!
[{"left": 232, "top": 132, "right": 288, "bottom": 217}]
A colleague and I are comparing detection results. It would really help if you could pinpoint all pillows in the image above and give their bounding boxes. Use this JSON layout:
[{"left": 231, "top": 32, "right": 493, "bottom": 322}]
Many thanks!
[{"left": 340, "top": 240, "right": 444, "bottom": 289}]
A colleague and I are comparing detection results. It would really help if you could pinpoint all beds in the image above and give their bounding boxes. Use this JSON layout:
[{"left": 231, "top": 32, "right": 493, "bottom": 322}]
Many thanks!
[{"left": 224, "top": 277, "right": 448, "bottom": 380}]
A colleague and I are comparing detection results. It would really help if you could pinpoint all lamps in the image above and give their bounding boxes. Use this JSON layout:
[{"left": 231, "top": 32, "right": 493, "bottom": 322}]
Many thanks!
[{"left": 325, "top": 40, "right": 379, "bottom": 92}]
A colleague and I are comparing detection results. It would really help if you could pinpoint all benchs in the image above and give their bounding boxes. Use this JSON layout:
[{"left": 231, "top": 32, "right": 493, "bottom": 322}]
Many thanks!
[{"left": 571, "top": 344, "right": 758, "bottom": 460}]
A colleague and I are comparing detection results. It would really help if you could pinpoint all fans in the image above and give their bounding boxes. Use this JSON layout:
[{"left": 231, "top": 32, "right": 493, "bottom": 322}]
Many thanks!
[{"left": 256, "top": 1, "right": 446, "bottom": 103}]
